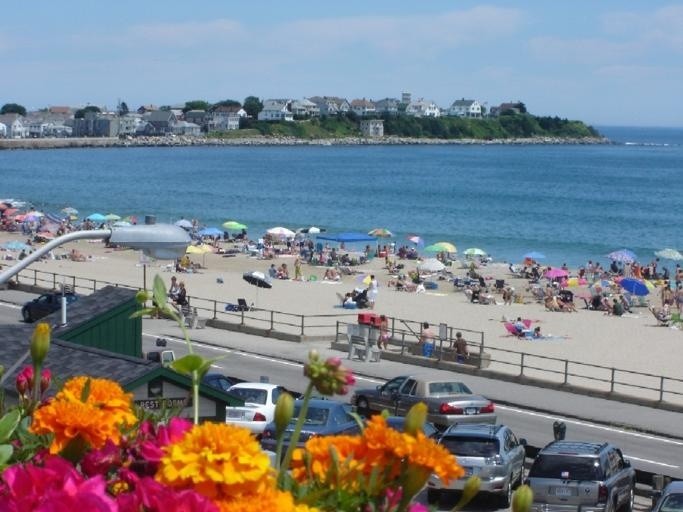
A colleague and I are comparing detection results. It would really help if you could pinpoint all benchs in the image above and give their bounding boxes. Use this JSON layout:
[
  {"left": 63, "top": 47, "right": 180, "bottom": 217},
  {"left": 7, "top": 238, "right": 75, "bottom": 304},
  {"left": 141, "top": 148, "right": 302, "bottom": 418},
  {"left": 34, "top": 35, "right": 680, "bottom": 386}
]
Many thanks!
[
  {"left": 346, "top": 343, "right": 381, "bottom": 362},
  {"left": 183, "top": 315, "right": 207, "bottom": 329}
]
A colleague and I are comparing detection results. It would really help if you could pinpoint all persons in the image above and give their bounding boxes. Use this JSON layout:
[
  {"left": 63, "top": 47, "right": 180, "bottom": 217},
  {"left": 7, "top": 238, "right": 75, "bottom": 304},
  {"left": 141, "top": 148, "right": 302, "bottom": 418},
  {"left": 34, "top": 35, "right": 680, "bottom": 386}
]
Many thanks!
[
  {"left": 342, "top": 197, "right": 683, "bottom": 368},
  {"left": 0, "top": 198, "right": 342, "bottom": 319}
]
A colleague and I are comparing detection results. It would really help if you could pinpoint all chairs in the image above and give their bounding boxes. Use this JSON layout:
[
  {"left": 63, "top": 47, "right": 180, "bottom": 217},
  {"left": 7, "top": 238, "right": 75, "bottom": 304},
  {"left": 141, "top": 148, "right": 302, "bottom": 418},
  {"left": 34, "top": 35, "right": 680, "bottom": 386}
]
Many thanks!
[
  {"left": 453, "top": 276, "right": 464, "bottom": 290},
  {"left": 649, "top": 306, "right": 672, "bottom": 326},
  {"left": 335, "top": 292, "right": 347, "bottom": 306},
  {"left": 583, "top": 297, "right": 592, "bottom": 309},
  {"left": 236, "top": 298, "right": 249, "bottom": 311}
]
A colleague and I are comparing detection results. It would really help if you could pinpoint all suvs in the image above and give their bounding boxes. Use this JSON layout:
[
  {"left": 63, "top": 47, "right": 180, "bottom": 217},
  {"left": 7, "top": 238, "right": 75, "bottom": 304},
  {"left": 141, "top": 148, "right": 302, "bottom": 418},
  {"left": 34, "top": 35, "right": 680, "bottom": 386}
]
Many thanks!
[
  {"left": 526, "top": 440, "right": 636, "bottom": 512},
  {"left": 426, "top": 423, "right": 529, "bottom": 509}
]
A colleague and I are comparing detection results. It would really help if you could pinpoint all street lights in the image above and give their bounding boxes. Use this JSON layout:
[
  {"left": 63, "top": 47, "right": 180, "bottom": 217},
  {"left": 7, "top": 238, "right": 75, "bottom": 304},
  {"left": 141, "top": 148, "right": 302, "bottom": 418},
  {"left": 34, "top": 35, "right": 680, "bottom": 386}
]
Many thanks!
[{"left": 1, "top": 224, "right": 192, "bottom": 279}]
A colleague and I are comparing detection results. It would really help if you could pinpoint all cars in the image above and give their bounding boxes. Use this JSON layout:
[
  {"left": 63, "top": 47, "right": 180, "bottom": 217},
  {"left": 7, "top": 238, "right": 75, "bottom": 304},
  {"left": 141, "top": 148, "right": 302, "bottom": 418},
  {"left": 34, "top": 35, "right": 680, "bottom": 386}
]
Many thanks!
[
  {"left": 647, "top": 481, "right": 683, "bottom": 512},
  {"left": 381, "top": 416, "right": 440, "bottom": 443},
  {"left": 353, "top": 376, "right": 497, "bottom": 426},
  {"left": 224, "top": 382, "right": 295, "bottom": 436},
  {"left": 22, "top": 290, "right": 81, "bottom": 323},
  {"left": 259, "top": 400, "right": 366, "bottom": 455}
]
